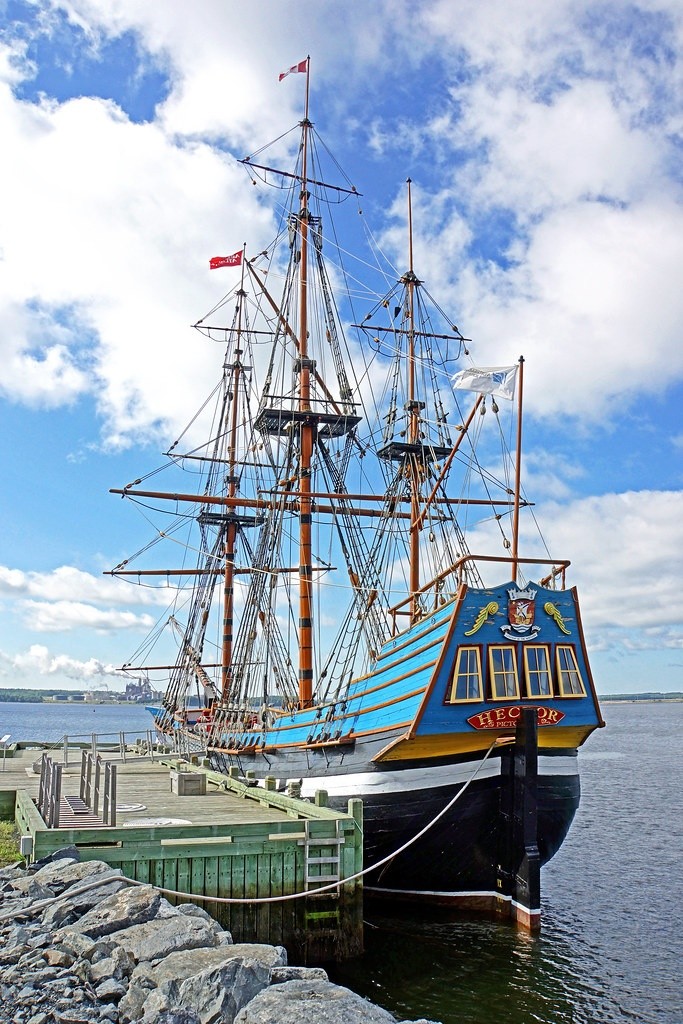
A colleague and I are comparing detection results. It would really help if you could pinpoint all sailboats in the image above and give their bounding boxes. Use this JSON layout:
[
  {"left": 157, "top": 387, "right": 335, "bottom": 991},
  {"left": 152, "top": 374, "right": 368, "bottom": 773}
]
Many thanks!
[{"left": 99, "top": 54, "right": 615, "bottom": 926}]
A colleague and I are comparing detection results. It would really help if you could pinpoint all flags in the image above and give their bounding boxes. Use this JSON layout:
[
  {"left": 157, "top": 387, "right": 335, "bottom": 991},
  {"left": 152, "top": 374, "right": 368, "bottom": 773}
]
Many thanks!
[
  {"left": 210, "top": 249, "right": 242, "bottom": 268},
  {"left": 279, "top": 60, "right": 306, "bottom": 82},
  {"left": 452, "top": 364, "right": 516, "bottom": 401}
]
